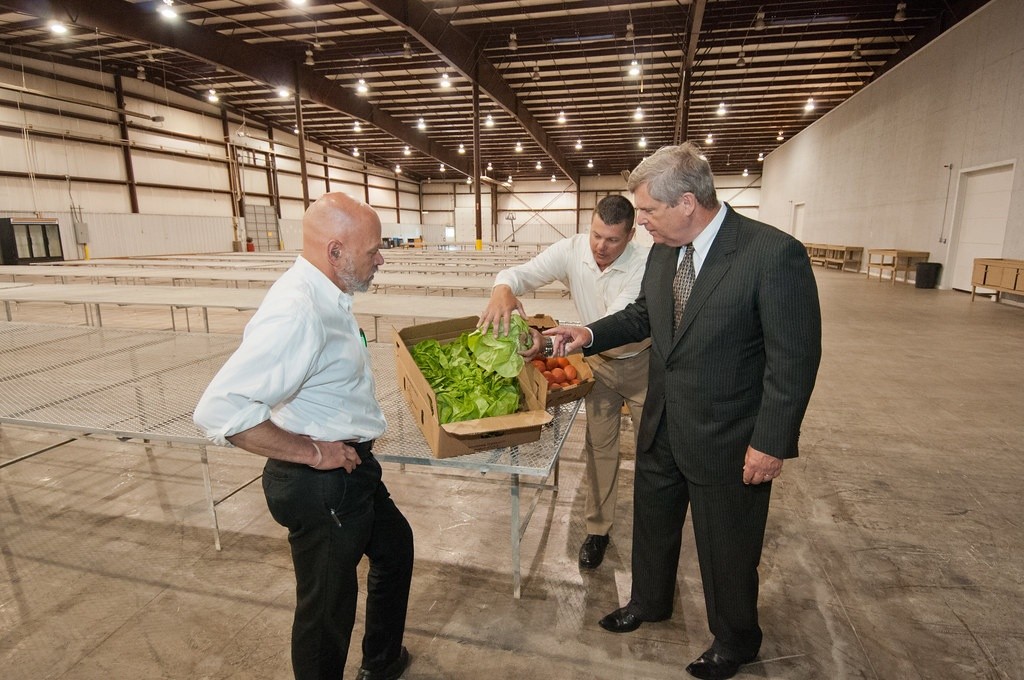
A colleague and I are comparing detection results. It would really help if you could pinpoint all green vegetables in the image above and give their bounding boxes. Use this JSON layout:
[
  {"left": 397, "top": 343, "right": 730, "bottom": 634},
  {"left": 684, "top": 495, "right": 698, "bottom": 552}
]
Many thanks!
[{"left": 410, "top": 314, "right": 533, "bottom": 425}]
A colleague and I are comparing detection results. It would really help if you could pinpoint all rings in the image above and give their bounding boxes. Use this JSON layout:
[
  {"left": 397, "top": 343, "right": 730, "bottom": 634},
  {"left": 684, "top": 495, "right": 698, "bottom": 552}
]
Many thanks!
[{"left": 766, "top": 474, "right": 774, "bottom": 477}]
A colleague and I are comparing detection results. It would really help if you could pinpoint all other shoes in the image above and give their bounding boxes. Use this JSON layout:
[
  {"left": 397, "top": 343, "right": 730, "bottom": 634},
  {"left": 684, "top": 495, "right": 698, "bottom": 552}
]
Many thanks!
[{"left": 355, "top": 645, "right": 408, "bottom": 680}]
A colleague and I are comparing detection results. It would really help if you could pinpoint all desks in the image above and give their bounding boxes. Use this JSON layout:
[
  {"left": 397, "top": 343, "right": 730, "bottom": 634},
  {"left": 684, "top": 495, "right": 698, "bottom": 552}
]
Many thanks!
[
  {"left": 866, "top": 248, "right": 930, "bottom": 285},
  {"left": 811, "top": 244, "right": 828, "bottom": 269},
  {"left": 803, "top": 242, "right": 814, "bottom": 264},
  {"left": 0, "top": 242, "right": 583, "bottom": 603},
  {"left": 971, "top": 258, "right": 1024, "bottom": 303},
  {"left": 826, "top": 245, "right": 864, "bottom": 272}
]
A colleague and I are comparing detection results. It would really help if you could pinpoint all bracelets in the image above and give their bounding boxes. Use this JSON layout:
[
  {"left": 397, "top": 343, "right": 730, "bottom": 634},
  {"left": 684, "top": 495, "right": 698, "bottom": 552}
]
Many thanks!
[
  {"left": 540, "top": 337, "right": 553, "bottom": 357},
  {"left": 310, "top": 441, "right": 322, "bottom": 467}
]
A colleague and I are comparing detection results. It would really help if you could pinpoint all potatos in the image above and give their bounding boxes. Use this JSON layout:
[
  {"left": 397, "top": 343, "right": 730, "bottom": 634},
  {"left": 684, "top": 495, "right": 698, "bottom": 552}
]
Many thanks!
[{"left": 529, "top": 356, "right": 580, "bottom": 392}]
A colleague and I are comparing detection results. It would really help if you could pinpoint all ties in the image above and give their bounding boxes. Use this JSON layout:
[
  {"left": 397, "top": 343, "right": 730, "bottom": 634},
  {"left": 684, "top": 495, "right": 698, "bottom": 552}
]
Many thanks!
[{"left": 672, "top": 241, "right": 695, "bottom": 339}]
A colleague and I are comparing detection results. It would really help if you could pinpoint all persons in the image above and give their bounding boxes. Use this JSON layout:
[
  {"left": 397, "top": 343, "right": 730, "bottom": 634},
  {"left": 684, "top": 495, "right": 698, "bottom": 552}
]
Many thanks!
[
  {"left": 475, "top": 194, "right": 652, "bottom": 569},
  {"left": 190, "top": 192, "right": 415, "bottom": 680},
  {"left": 538, "top": 144, "right": 822, "bottom": 680}
]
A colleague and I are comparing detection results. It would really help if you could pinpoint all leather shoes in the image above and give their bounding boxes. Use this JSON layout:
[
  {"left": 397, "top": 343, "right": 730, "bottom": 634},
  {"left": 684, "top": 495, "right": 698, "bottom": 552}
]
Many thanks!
[
  {"left": 578, "top": 533, "right": 610, "bottom": 569},
  {"left": 685, "top": 646, "right": 743, "bottom": 680},
  {"left": 598, "top": 606, "right": 642, "bottom": 633}
]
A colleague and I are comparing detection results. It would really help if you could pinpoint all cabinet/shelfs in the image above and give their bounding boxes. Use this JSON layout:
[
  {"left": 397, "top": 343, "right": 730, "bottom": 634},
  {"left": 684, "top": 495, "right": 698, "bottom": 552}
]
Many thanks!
[{"left": 407, "top": 238, "right": 422, "bottom": 248}]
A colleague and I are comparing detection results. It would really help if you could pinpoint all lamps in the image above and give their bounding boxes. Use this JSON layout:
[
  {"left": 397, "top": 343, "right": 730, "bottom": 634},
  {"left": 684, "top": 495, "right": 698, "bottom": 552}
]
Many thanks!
[
  {"left": 777, "top": 130, "right": 784, "bottom": 141},
  {"left": 754, "top": 12, "right": 766, "bottom": 31},
  {"left": 46, "top": 0, "right": 567, "bottom": 184},
  {"left": 706, "top": 133, "right": 714, "bottom": 144},
  {"left": 587, "top": 160, "right": 593, "bottom": 168},
  {"left": 628, "top": 60, "right": 640, "bottom": 75},
  {"left": 575, "top": 140, "right": 582, "bottom": 149},
  {"left": 757, "top": 153, "right": 764, "bottom": 161},
  {"left": 893, "top": 3, "right": 907, "bottom": 21},
  {"left": 851, "top": 45, "right": 862, "bottom": 59},
  {"left": 805, "top": 97, "right": 814, "bottom": 110},
  {"left": 736, "top": 52, "right": 746, "bottom": 67},
  {"left": 625, "top": 23, "right": 636, "bottom": 42},
  {"left": 639, "top": 137, "right": 647, "bottom": 147},
  {"left": 742, "top": 169, "right": 748, "bottom": 177},
  {"left": 634, "top": 107, "right": 642, "bottom": 119},
  {"left": 717, "top": 102, "right": 726, "bottom": 115}
]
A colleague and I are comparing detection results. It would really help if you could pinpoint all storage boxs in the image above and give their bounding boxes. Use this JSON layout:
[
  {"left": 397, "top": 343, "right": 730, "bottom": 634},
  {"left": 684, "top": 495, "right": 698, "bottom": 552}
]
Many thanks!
[
  {"left": 391, "top": 314, "right": 555, "bottom": 457},
  {"left": 526, "top": 314, "right": 596, "bottom": 408}
]
